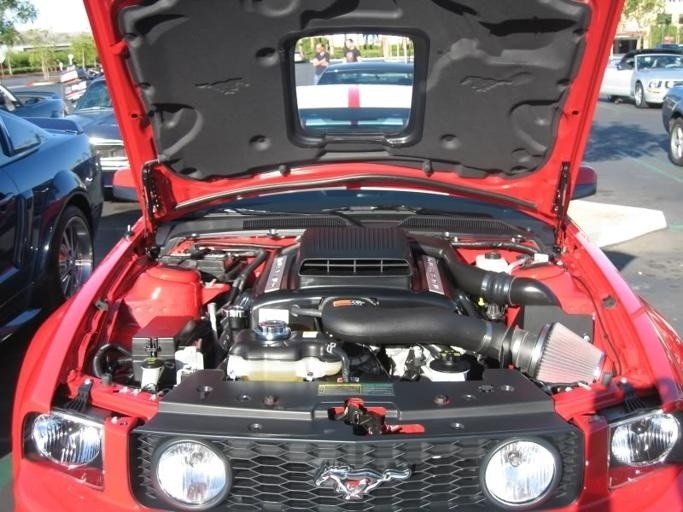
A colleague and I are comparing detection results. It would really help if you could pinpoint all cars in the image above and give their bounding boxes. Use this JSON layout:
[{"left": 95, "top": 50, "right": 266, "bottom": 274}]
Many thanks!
[
  {"left": 1, "top": 83, "right": 68, "bottom": 119},
  {"left": 294, "top": 53, "right": 305, "bottom": 63},
  {"left": 1, "top": 66, "right": 140, "bottom": 351},
  {"left": 601, "top": 44, "right": 682, "bottom": 164},
  {"left": 301, "top": 63, "right": 413, "bottom": 125},
  {"left": 64, "top": 75, "right": 130, "bottom": 188},
  {"left": 10, "top": 0, "right": 682, "bottom": 512}
]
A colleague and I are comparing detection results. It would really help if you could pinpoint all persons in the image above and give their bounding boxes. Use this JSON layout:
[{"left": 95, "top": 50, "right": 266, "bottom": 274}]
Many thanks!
[
  {"left": 341, "top": 38, "right": 362, "bottom": 65},
  {"left": 309, "top": 42, "right": 330, "bottom": 84}
]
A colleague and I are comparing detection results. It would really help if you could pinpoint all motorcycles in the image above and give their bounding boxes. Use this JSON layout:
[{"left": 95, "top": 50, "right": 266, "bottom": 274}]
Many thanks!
[{"left": 59, "top": 53, "right": 103, "bottom": 101}]
